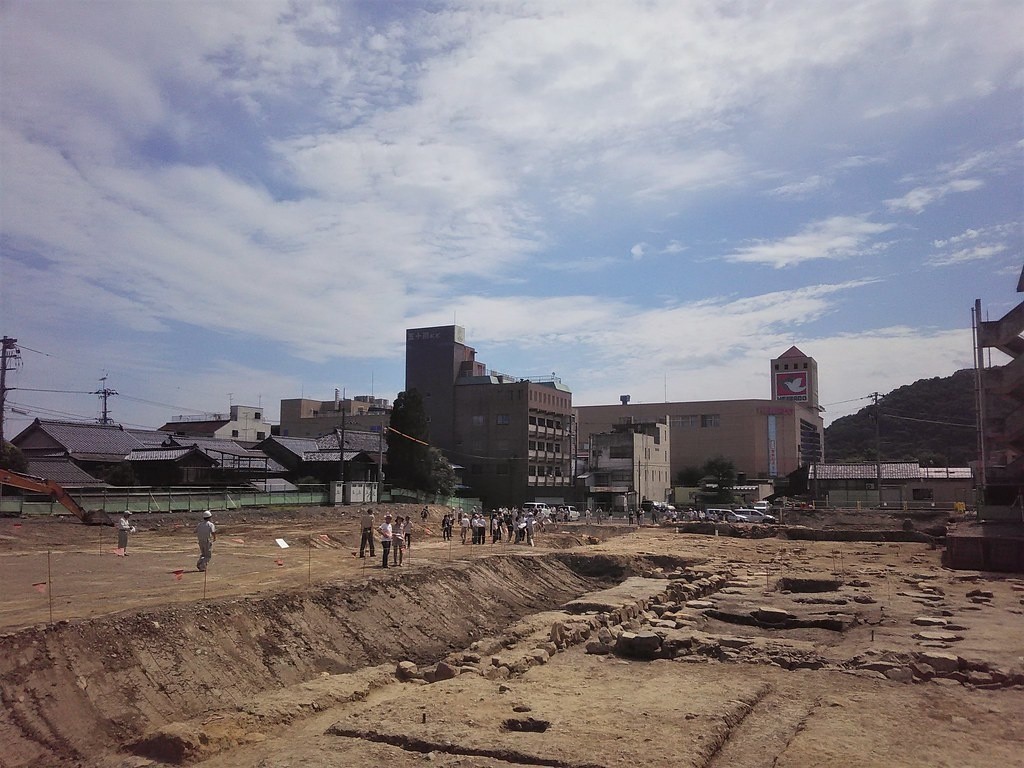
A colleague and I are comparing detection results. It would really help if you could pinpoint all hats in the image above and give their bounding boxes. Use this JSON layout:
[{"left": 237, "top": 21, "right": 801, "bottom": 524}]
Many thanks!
[
  {"left": 452, "top": 507, "right": 455, "bottom": 510},
  {"left": 528, "top": 512, "right": 533, "bottom": 516},
  {"left": 479, "top": 514, "right": 483, "bottom": 519},
  {"left": 474, "top": 514, "right": 479, "bottom": 518},
  {"left": 394, "top": 516, "right": 404, "bottom": 523},
  {"left": 539, "top": 509, "right": 542, "bottom": 511},
  {"left": 492, "top": 509, "right": 495, "bottom": 512},
  {"left": 124, "top": 511, "right": 133, "bottom": 516},
  {"left": 463, "top": 513, "right": 468, "bottom": 518}
]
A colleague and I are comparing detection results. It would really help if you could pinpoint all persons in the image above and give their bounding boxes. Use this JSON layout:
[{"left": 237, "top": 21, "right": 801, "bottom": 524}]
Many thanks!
[
  {"left": 596, "top": 507, "right": 602, "bottom": 525},
  {"left": 629, "top": 507, "right": 731, "bottom": 526},
  {"left": 421, "top": 506, "right": 430, "bottom": 522},
  {"left": 194, "top": 511, "right": 216, "bottom": 572},
  {"left": 360, "top": 508, "right": 376, "bottom": 558},
  {"left": 549, "top": 505, "right": 571, "bottom": 525},
  {"left": 442, "top": 506, "right": 546, "bottom": 545},
  {"left": 585, "top": 508, "right": 591, "bottom": 525},
  {"left": 608, "top": 508, "right": 613, "bottom": 525},
  {"left": 377, "top": 515, "right": 414, "bottom": 568},
  {"left": 954, "top": 499, "right": 962, "bottom": 514},
  {"left": 118, "top": 511, "right": 133, "bottom": 556}
]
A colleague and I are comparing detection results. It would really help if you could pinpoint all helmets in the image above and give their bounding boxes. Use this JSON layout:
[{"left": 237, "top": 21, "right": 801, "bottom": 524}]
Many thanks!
[{"left": 203, "top": 511, "right": 212, "bottom": 518}]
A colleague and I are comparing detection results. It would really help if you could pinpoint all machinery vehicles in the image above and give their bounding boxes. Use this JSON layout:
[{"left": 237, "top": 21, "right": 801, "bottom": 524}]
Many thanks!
[{"left": 0, "top": 469, "right": 114, "bottom": 526}]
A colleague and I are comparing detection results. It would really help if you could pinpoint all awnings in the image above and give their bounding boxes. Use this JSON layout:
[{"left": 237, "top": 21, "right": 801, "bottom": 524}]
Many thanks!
[
  {"left": 722, "top": 486, "right": 758, "bottom": 490},
  {"left": 577, "top": 473, "right": 593, "bottom": 479}
]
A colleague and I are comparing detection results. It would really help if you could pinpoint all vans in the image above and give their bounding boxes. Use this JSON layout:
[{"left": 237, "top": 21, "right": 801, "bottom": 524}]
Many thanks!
[
  {"left": 557, "top": 506, "right": 580, "bottom": 521},
  {"left": 522, "top": 503, "right": 551, "bottom": 517},
  {"left": 734, "top": 509, "right": 775, "bottom": 524},
  {"left": 641, "top": 501, "right": 660, "bottom": 511},
  {"left": 706, "top": 508, "right": 749, "bottom": 522}
]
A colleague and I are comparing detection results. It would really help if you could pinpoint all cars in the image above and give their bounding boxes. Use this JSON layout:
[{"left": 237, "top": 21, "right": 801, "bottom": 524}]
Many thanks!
[{"left": 754, "top": 501, "right": 773, "bottom": 513}]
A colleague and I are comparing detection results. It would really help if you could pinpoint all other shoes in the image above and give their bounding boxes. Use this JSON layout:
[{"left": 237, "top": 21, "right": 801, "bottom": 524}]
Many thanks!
[
  {"left": 197, "top": 561, "right": 200, "bottom": 569},
  {"left": 384, "top": 565, "right": 390, "bottom": 568},
  {"left": 393, "top": 562, "right": 398, "bottom": 566},
  {"left": 124, "top": 553, "right": 130, "bottom": 556},
  {"left": 400, "top": 563, "right": 403, "bottom": 567},
  {"left": 370, "top": 554, "right": 376, "bottom": 557},
  {"left": 360, "top": 556, "right": 365, "bottom": 558}
]
